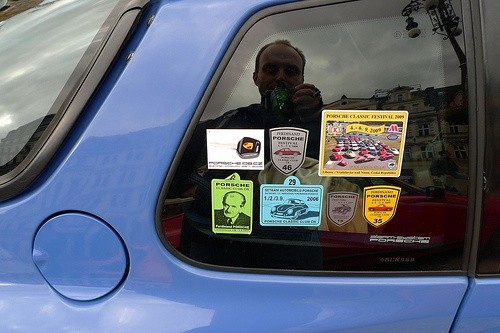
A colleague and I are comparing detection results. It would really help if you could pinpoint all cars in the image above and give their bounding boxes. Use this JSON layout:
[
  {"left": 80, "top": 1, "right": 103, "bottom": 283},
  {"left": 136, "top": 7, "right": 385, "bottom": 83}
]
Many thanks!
[
  {"left": 0, "top": 0, "right": 500, "bottom": 332},
  {"left": 314, "top": 173, "right": 495, "bottom": 255},
  {"left": 331, "top": 132, "right": 400, "bottom": 166}
]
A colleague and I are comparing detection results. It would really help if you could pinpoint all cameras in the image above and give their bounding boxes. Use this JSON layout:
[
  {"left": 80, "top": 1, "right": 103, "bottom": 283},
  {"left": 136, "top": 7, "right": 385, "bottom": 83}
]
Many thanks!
[{"left": 263, "top": 88, "right": 299, "bottom": 115}]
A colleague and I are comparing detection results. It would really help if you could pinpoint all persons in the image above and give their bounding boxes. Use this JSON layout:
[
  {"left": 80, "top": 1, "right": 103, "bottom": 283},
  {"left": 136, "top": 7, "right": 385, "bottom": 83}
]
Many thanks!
[
  {"left": 178, "top": 37, "right": 346, "bottom": 271},
  {"left": 431, "top": 150, "right": 458, "bottom": 190},
  {"left": 213, "top": 190, "right": 250, "bottom": 229}
]
